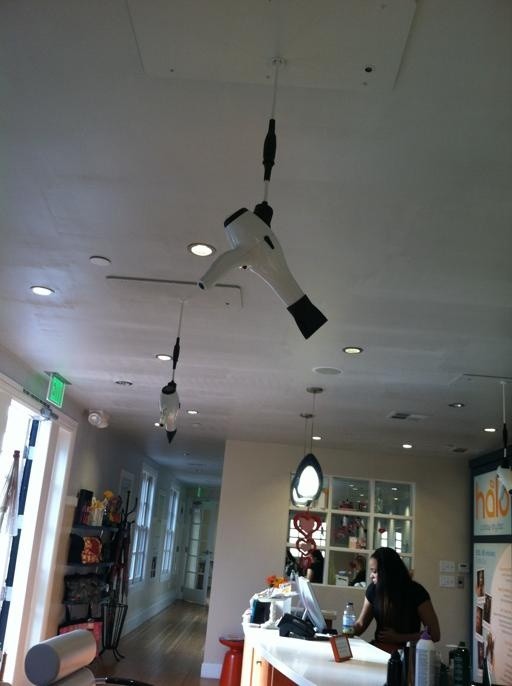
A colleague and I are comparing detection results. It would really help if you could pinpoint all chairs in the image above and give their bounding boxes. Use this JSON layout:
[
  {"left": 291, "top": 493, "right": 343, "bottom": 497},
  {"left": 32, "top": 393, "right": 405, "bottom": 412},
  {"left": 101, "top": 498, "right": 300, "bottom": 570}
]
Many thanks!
[{"left": 23, "top": 629, "right": 154, "bottom": 686}]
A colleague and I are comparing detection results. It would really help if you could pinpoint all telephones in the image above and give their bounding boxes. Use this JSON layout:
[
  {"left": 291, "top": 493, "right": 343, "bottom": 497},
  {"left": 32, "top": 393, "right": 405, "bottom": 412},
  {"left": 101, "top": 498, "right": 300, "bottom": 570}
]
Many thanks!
[{"left": 278, "top": 614, "right": 315, "bottom": 640}]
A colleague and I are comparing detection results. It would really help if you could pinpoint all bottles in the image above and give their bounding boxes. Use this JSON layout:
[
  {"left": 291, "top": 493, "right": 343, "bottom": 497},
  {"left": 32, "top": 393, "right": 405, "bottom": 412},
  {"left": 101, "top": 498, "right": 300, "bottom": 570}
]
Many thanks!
[
  {"left": 342, "top": 601, "right": 356, "bottom": 639},
  {"left": 388, "top": 624, "right": 489, "bottom": 686}
]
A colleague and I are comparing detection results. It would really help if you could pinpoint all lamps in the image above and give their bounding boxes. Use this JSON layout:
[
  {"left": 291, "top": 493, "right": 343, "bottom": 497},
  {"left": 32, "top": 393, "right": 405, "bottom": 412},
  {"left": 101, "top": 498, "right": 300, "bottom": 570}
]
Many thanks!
[{"left": 291, "top": 384, "right": 324, "bottom": 511}]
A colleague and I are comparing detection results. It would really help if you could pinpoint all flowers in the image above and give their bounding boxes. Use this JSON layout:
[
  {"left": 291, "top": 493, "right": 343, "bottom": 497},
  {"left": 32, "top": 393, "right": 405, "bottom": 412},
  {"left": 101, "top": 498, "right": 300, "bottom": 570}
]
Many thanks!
[{"left": 87, "top": 490, "right": 113, "bottom": 511}]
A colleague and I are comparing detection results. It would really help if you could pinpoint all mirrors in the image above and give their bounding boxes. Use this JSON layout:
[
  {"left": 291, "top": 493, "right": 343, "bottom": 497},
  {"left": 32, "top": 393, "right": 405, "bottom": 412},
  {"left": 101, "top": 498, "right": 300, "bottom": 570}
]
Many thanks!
[{"left": 283, "top": 472, "right": 413, "bottom": 591}]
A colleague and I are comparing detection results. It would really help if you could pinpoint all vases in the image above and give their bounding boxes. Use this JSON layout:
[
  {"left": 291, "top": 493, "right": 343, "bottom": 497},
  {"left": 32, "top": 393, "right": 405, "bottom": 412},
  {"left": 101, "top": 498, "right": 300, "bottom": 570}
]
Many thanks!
[{"left": 86, "top": 510, "right": 103, "bottom": 527}]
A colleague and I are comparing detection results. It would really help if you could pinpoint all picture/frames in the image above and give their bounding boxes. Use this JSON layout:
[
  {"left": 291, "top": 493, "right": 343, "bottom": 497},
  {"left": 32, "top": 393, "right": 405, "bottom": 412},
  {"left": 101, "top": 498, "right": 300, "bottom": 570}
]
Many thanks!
[{"left": 330, "top": 634, "right": 351, "bottom": 662}]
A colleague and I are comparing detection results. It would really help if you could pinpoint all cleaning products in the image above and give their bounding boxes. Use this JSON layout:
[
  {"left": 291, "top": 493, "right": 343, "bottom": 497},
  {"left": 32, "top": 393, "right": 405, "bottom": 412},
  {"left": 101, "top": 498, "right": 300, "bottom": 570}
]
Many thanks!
[
  {"left": 414, "top": 627, "right": 437, "bottom": 685},
  {"left": 341, "top": 601, "right": 357, "bottom": 637},
  {"left": 448, "top": 641, "right": 468, "bottom": 683}
]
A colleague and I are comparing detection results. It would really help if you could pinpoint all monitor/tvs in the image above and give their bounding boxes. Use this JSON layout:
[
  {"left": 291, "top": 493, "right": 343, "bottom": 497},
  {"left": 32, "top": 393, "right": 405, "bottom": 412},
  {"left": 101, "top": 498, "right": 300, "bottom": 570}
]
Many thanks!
[{"left": 297, "top": 576, "right": 328, "bottom": 632}]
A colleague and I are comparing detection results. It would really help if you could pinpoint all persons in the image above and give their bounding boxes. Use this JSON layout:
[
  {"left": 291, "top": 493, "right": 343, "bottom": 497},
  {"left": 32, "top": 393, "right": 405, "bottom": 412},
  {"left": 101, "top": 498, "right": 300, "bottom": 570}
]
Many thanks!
[
  {"left": 306, "top": 550, "right": 324, "bottom": 583},
  {"left": 477, "top": 570, "right": 484, "bottom": 597},
  {"left": 484, "top": 596, "right": 490, "bottom": 622},
  {"left": 476, "top": 607, "right": 482, "bottom": 634},
  {"left": 479, "top": 644, "right": 484, "bottom": 669},
  {"left": 355, "top": 547, "right": 441, "bottom": 686},
  {"left": 349, "top": 556, "right": 366, "bottom": 586}
]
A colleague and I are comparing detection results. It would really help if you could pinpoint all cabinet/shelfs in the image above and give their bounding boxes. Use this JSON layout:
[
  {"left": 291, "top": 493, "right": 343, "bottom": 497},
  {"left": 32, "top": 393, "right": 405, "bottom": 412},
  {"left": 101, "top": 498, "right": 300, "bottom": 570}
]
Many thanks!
[{"left": 61, "top": 527, "right": 129, "bottom": 623}]
